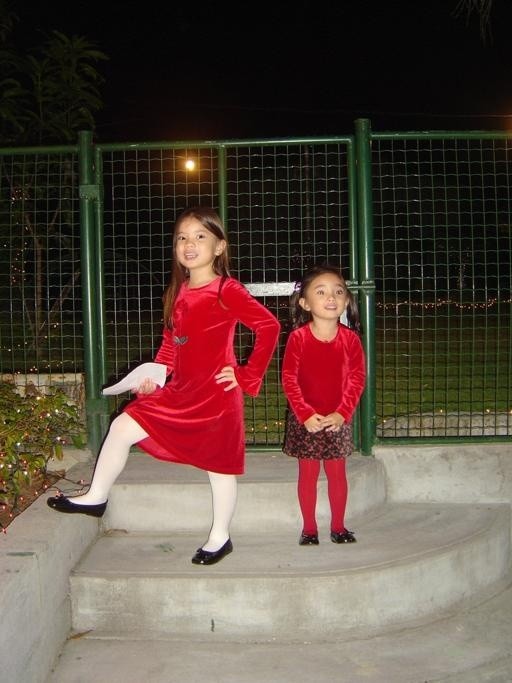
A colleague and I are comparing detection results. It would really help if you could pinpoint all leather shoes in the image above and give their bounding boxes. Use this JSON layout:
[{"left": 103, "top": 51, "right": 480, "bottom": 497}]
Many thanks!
[
  {"left": 192, "top": 537, "right": 233, "bottom": 566},
  {"left": 299, "top": 528, "right": 320, "bottom": 544},
  {"left": 47, "top": 493, "right": 109, "bottom": 518},
  {"left": 329, "top": 528, "right": 355, "bottom": 543}
]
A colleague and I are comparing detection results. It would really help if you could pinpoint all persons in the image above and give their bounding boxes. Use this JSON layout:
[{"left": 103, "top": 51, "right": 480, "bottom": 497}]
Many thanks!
[
  {"left": 281, "top": 266, "right": 368, "bottom": 546},
  {"left": 46, "top": 206, "right": 281, "bottom": 564}
]
